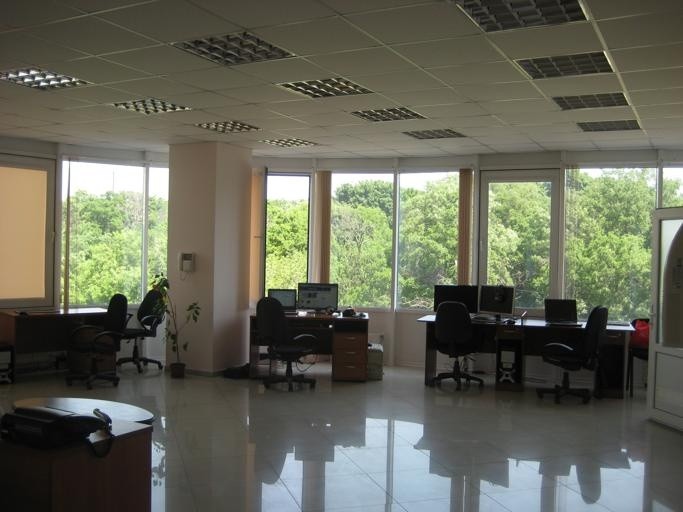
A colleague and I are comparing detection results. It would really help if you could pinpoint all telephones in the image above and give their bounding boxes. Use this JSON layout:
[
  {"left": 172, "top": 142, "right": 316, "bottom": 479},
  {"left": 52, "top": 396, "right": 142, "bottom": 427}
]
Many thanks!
[
  {"left": 178, "top": 251, "right": 194, "bottom": 272},
  {"left": 0, "top": 406, "right": 105, "bottom": 449}
]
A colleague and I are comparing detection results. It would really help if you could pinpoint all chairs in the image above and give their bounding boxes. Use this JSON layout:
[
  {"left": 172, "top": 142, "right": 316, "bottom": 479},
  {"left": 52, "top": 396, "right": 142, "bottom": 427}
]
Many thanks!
[
  {"left": 115, "top": 288, "right": 164, "bottom": 373},
  {"left": 64, "top": 294, "right": 127, "bottom": 391},
  {"left": 256, "top": 297, "right": 319, "bottom": 393},
  {"left": 536, "top": 305, "right": 609, "bottom": 405},
  {"left": 626, "top": 317, "right": 650, "bottom": 398},
  {"left": 429, "top": 301, "right": 485, "bottom": 393}
]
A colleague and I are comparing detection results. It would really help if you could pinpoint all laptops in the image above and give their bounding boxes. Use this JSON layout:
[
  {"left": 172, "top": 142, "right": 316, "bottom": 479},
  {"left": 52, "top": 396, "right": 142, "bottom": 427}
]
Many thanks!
[
  {"left": 544, "top": 298, "right": 582, "bottom": 328},
  {"left": 268, "top": 289, "right": 298, "bottom": 315}
]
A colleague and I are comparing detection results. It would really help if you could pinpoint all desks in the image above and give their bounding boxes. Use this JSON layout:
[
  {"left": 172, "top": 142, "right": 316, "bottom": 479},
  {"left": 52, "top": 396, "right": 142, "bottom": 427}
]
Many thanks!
[
  {"left": 250, "top": 309, "right": 369, "bottom": 383},
  {"left": 12, "top": 396, "right": 156, "bottom": 425},
  {"left": 0, "top": 307, "right": 120, "bottom": 386},
  {"left": 416, "top": 314, "right": 636, "bottom": 399},
  {"left": 0, "top": 419, "right": 154, "bottom": 512}
]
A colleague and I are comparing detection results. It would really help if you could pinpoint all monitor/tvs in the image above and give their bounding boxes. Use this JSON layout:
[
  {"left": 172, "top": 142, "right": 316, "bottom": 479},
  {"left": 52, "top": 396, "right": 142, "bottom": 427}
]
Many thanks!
[
  {"left": 478, "top": 285, "right": 516, "bottom": 324},
  {"left": 433, "top": 284, "right": 480, "bottom": 314},
  {"left": 297, "top": 282, "right": 338, "bottom": 314}
]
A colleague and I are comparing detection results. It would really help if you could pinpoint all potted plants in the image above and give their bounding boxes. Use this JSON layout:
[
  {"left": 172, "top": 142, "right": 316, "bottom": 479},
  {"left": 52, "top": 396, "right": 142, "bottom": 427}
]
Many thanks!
[{"left": 150, "top": 271, "right": 200, "bottom": 379}]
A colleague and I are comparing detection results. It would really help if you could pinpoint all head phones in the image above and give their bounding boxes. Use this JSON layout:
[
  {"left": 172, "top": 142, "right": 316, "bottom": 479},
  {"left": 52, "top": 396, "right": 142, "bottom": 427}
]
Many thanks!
[
  {"left": 493, "top": 283, "right": 506, "bottom": 303},
  {"left": 325, "top": 306, "right": 342, "bottom": 314}
]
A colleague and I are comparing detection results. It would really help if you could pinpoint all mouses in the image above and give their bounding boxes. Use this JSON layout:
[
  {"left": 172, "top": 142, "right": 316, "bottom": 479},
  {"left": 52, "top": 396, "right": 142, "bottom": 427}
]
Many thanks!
[{"left": 504, "top": 319, "right": 516, "bottom": 324}]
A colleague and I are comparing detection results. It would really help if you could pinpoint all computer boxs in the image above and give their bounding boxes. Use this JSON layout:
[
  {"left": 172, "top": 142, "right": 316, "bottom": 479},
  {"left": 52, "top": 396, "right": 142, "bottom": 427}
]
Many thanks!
[{"left": 495, "top": 335, "right": 523, "bottom": 385}]
